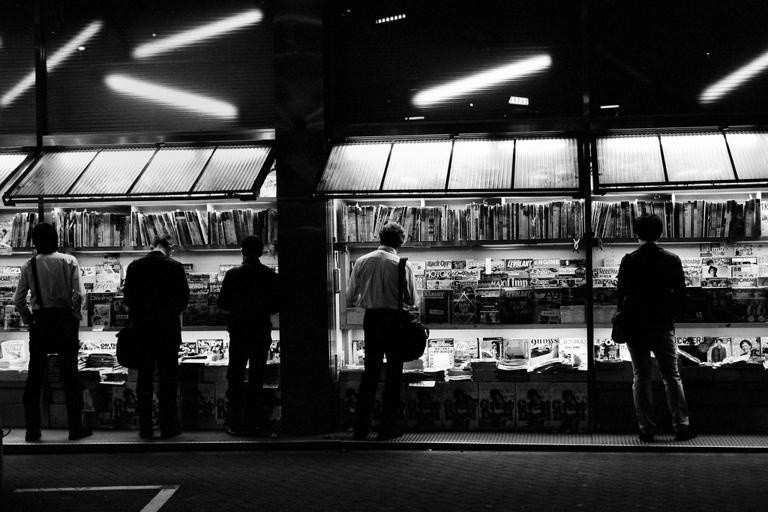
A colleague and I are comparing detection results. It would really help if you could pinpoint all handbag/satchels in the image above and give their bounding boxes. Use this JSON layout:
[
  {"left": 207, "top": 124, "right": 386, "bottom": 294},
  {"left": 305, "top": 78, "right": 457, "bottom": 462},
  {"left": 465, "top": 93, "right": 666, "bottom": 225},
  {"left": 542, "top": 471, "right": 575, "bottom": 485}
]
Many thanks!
[
  {"left": 40, "top": 310, "right": 77, "bottom": 353},
  {"left": 612, "top": 310, "right": 626, "bottom": 343},
  {"left": 396, "top": 307, "right": 426, "bottom": 362},
  {"left": 117, "top": 321, "right": 139, "bottom": 369}
]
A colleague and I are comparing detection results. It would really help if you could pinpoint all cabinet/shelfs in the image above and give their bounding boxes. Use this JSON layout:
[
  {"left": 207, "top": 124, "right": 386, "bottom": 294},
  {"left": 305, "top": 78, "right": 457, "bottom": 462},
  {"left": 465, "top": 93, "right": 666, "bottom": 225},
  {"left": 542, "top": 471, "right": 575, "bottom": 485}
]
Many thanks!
[
  {"left": 0, "top": 195, "right": 279, "bottom": 430},
  {"left": 321, "top": 184, "right": 768, "bottom": 432}
]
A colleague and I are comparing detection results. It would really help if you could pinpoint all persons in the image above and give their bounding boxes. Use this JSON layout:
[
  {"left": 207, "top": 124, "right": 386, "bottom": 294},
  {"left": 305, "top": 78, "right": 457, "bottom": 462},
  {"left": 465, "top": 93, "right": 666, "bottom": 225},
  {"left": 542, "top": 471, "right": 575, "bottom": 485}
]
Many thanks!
[
  {"left": 216, "top": 237, "right": 284, "bottom": 437},
  {"left": 612, "top": 213, "right": 699, "bottom": 442},
  {"left": 339, "top": 386, "right": 589, "bottom": 432},
  {"left": 348, "top": 223, "right": 417, "bottom": 440},
  {"left": 111, "top": 388, "right": 282, "bottom": 429},
  {"left": 11, "top": 220, "right": 92, "bottom": 443},
  {"left": 122, "top": 233, "right": 192, "bottom": 441}
]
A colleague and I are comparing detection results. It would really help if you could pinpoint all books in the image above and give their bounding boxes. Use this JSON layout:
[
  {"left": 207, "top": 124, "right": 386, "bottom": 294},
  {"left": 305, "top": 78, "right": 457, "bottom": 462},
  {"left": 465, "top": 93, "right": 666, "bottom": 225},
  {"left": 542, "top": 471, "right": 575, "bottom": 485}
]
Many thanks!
[
  {"left": 682, "top": 242, "right": 768, "bottom": 321},
  {"left": 0, "top": 340, "right": 29, "bottom": 379},
  {"left": 0, "top": 266, "right": 32, "bottom": 332},
  {"left": 78, "top": 341, "right": 128, "bottom": 379},
  {"left": 674, "top": 336, "right": 768, "bottom": 380},
  {"left": 591, "top": 193, "right": 768, "bottom": 237},
  {"left": 79, "top": 263, "right": 129, "bottom": 327},
  {"left": 404, "top": 337, "right": 633, "bottom": 379},
  {"left": 348, "top": 263, "right": 366, "bottom": 367},
  {"left": 1, "top": 209, "right": 278, "bottom": 248},
  {"left": 178, "top": 339, "right": 279, "bottom": 380},
  {"left": 340, "top": 200, "right": 586, "bottom": 242},
  {"left": 182, "top": 262, "right": 278, "bottom": 313},
  {"left": 411, "top": 258, "right": 621, "bottom": 324}
]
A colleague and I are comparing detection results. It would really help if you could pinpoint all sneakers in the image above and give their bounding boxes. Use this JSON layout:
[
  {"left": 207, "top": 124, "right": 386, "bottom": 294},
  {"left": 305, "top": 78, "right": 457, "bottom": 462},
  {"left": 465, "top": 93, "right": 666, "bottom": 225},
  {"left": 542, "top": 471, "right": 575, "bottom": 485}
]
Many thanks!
[
  {"left": 26, "top": 430, "right": 41, "bottom": 442},
  {"left": 70, "top": 428, "right": 92, "bottom": 439},
  {"left": 639, "top": 430, "right": 696, "bottom": 442}
]
